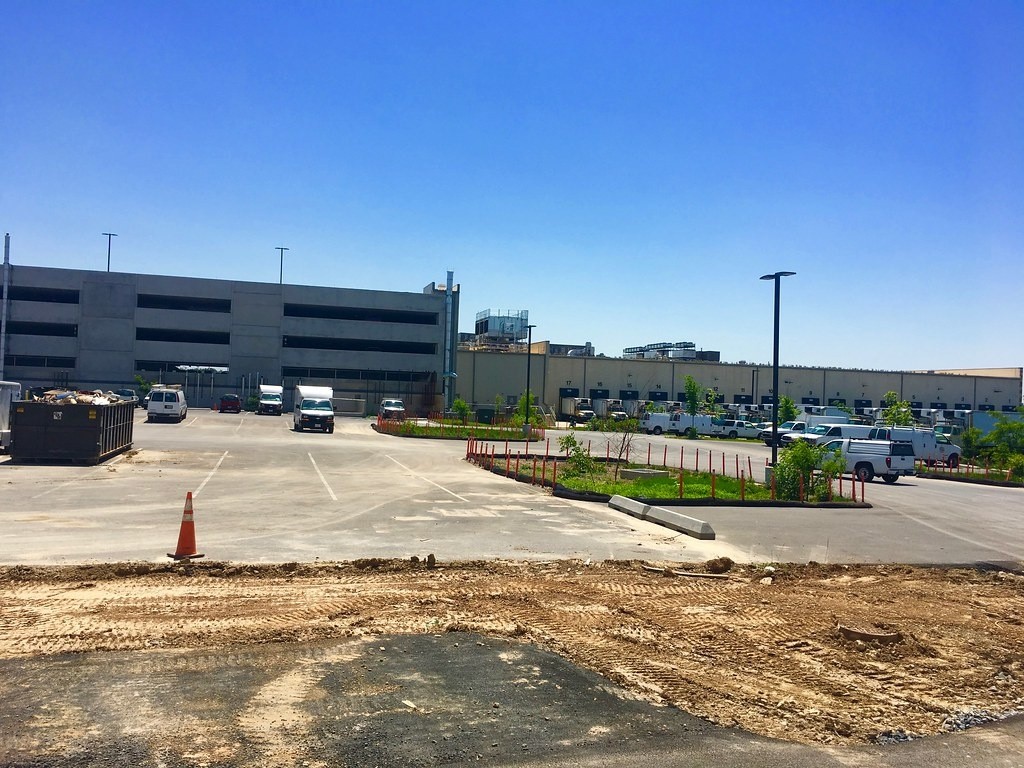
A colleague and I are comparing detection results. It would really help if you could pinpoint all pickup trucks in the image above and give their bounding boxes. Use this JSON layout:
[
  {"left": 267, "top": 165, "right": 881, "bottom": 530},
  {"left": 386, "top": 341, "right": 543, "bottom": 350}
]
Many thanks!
[{"left": 762, "top": 416, "right": 963, "bottom": 483}]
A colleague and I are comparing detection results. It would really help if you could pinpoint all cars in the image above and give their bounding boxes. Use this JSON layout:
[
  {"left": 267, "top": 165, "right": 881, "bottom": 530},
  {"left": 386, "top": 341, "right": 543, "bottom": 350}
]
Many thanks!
[
  {"left": 380, "top": 398, "right": 405, "bottom": 422},
  {"left": 219, "top": 394, "right": 243, "bottom": 413},
  {"left": 710, "top": 419, "right": 764, "bottom": 440}
]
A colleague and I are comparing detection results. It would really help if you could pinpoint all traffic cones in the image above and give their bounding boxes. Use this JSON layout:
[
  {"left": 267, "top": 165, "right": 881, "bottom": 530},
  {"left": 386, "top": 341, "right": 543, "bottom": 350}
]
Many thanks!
[{"left": 166, "top": 491, "right": 205, "bottom": 559}]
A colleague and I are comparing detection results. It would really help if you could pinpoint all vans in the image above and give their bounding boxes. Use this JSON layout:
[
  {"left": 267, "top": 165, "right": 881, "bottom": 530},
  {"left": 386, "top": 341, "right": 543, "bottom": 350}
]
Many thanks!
[{"left": 147, "top": 388, "right": 188, "bottom": 423}]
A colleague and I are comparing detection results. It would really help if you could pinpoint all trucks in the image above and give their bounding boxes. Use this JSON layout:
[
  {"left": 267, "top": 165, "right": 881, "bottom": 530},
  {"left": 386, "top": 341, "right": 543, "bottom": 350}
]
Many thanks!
[
  {"left": 256, "top": 385, "right": 284, "bottom": 415},
  {"left": 556, "top": 398, "right": 1024, "bottom": 449},
  {"left": 294, "top": 385, "right": 338, "bottom": 433}
]
